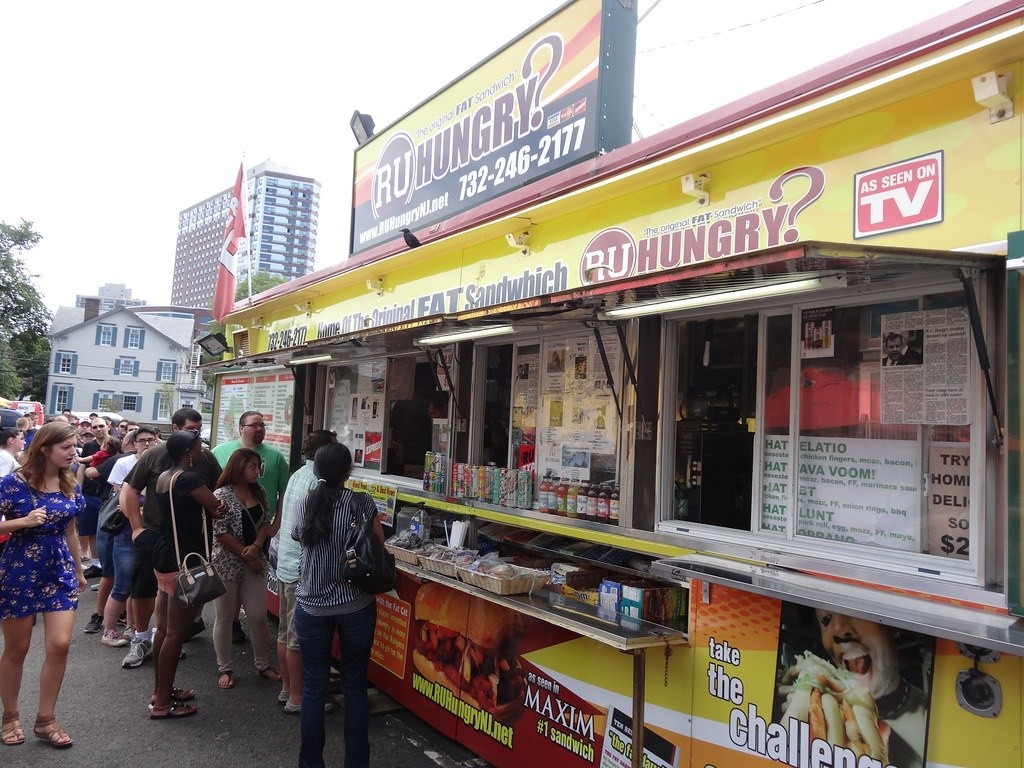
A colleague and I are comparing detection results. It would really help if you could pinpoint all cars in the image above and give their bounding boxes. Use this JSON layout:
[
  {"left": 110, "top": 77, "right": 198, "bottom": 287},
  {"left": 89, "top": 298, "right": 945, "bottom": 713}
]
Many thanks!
[
  {"left": 199, "top": 428, "right": 211, "bottom": 445},
  {"left": 0, "top": 409, "right": 43, "bottom": 442}
]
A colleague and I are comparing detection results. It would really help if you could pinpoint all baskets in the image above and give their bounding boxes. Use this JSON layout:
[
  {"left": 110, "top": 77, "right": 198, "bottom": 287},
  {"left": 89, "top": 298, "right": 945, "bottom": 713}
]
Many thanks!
[{"left": 384, "top": 530, "right": 548, "bottom": 596}]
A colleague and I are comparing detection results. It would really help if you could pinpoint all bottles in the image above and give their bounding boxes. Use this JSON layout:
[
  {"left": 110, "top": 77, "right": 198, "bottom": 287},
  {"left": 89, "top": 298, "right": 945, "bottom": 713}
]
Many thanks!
[
  {"left": 396, "top": 507, "right": 431, "bottom": 543},
  {"left": 114, "top": 428, "right": 119, "bottom": 438},
  {"left": 539, "top": 476, "right": 619, "bottom": 526},
  {"left": 488, "top": 462, "right": 497, "bottom": 468},
  {"left": 352, "top": 401, "right": 357, "bottom": 417}
]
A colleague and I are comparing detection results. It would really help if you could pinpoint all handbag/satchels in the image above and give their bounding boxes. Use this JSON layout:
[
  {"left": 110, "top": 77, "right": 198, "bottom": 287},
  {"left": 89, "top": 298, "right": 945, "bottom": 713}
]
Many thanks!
[
  {"left": 341, "top": 514, "right": 399, "bottom": 594},
  {"left": 174, "top": 551, "right": 227, "bottom": 609}
]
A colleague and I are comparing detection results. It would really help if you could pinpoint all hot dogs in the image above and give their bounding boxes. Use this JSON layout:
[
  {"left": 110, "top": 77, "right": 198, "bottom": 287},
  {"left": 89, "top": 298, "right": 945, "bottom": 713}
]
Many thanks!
[{"left": 776, "top": 651, "right": 888, "bottom": 768}]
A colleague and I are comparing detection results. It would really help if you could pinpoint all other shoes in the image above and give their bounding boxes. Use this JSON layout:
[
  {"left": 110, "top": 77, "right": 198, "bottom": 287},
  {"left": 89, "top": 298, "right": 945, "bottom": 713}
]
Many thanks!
[
  {"left": 191, "top": 618, "right": 205, "bottom": 636},
  {"left": 232, "top": 619, "right": 246, "bottom": 643},
  {"left": 82, "top": 565, "right": 103, "bottom": 578}
]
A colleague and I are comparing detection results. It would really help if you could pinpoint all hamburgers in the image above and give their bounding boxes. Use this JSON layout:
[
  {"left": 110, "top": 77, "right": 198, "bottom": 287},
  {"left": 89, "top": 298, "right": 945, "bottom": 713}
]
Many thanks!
[{"left": 412, "top": 583, "right": 526, "bottom": 724}]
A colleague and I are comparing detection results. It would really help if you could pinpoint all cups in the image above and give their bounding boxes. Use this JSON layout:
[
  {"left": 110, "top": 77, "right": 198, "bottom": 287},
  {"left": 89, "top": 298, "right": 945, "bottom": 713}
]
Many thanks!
[
  {"left": 805, "top": 320, "right": 832, "bottom": 350},
  {"left": 549, "top": 397, "right": 563, "bottom": 426},
  {"left": 572, "top": 393, "right": 584, "bottom": 425}
]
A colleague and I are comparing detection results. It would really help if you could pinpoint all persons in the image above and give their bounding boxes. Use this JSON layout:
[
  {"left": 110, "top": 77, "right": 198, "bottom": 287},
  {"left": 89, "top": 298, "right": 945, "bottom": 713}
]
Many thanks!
[
  {"left": 147, "top": 429, "right": 230, "bottom": 718},
  {"left": 211, "top": 447, "right": 281, "bottom": 688},
  {"left": 548, "top": 353, "right": 564, "bottom": 372},
  {"left": 597, "top": 409, "right": 605, "bottom": 429},
  {"left": 0, "top": 422, "right": 88, "bottom": 749},
  {"left": 562, "top": 452, "right": 587, "bottom": 468},
  {"left": 211, "top": 411, "right": 287, "bottom": 643},
  {"left": 883, "top": 333, "right": 923, "bottom": 366},
  {"left": 290, "top": 443, "right": 384, "bottom": 768},
  {"left": 580, "top": 363, "right": 585, "bottom": 379},
  {"left": 121, "top": 408, "right": 223, "bottom": 668},
  {"left": 84, "top": 426, "right": 158, "bottom": 647},
  {"left": 275, "top": 429, "right": 335, "bottom": 714},
  {"left": 778, "top": 608, "right": 928, "bottom": 768},
  {"left": 0, "top": 408, "right": 168, "bottom": 594}
]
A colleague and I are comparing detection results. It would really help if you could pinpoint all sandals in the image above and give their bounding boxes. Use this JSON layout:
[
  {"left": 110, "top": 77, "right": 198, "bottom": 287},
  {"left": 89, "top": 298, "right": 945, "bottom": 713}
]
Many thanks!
[
  {"left": 218, "top": 671, "right": 235, "bottom": 689},
  {"left": 34, "top": 713, "right": 74, "bottom": 749},
  {"left": 255, "top": 667, "right": 282, "bottom": 680},
  {"left": 0, "top": 711, "right": 26, "bottom": 745}
]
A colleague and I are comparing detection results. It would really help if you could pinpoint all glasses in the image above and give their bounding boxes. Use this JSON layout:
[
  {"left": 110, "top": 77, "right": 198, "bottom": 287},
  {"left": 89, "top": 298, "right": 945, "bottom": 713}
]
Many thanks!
[
  {"left": 137, "top": 438, "right": 156, "bottom": 444},
  {"left": 241, "top": 423, "right": 266, "bottom": 428},
  {"left": 91, "top": 425, "right": 105, "bottom": 429},
  {"left": 121, "top": 425, "right": 126, "bottom": 428}
]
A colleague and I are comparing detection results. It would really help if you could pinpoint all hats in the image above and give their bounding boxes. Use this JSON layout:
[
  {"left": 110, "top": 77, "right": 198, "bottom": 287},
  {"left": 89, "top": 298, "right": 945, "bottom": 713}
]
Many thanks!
[{"left": 78, "top": 417, "right": 92, "bottom": 425}]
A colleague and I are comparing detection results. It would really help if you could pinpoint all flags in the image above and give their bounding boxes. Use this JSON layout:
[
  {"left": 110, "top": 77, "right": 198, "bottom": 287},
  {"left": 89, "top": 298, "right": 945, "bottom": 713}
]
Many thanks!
[{"left": 212, "top": 161, "right": 249, "bottom": 325}]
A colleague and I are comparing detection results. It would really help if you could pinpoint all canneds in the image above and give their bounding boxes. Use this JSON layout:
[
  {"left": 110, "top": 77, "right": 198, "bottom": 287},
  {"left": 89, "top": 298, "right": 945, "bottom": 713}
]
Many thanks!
[
  {"left": 422, "top": 451, "right": 446, "bottom": 495},
  {"left": 452, "top": 462, "right": 533, "bottom": 510}
]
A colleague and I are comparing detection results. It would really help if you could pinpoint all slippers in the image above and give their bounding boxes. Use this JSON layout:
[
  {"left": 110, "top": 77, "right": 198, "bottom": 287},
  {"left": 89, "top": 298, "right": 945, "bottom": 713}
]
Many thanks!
[{"left": 150, "top": 688, "right": 198, "bottom": 718}]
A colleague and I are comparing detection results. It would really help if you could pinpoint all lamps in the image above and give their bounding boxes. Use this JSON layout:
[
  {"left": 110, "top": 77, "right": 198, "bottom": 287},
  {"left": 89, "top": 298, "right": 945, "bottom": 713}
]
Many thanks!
[
  {"left": 597, "top": 274, "right": 848, "bottom": 322},
  {"left": 294, "top": 300, "right": 313, "bottom": 318},
  {"left": 972, "top": 72, "right": 1014, "bottom": 123},
  {"left": 194, "top": 333, "right": 233, "bottom": 357},
  {"left": 414, "top": 324, "right": 538, "bottom": 346},
  {"left": 366, "top": 276, "right": 384, "bottom": 296},
  {"left": 680, "top": 170, "right": 711, "bottom": 210},
  {"left": 506, "top": 230, "right": 530, "bottom": 257},
  {"left": 350, "top": 110, "right": 375, "bottom": 145},
  {"left": 289, "top": 351, "right": 350, "bottom": 366}
]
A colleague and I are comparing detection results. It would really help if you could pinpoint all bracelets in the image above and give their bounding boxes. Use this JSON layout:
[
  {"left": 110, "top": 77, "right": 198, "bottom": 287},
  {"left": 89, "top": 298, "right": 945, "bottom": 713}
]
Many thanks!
[{"left": 253, "top": 543, "right": 260, "bottom": 553}]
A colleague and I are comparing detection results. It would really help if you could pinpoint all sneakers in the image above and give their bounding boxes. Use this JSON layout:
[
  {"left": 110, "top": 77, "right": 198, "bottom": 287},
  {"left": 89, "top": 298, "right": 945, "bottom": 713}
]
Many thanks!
[
  {"left": 101, "top": 629, "right": 128, "bottom": 647},
  {"left": 117, "top": 611, "right": 127, "bottom": 626},
  {"left": 278, "top": 690, "right": 334, "bottom": 714},
  {"left": 121, "top": 638, "right": 154, "bottom": 668},
  {"left": 83, "top": 613, "right": 103, "bottom": 633}
]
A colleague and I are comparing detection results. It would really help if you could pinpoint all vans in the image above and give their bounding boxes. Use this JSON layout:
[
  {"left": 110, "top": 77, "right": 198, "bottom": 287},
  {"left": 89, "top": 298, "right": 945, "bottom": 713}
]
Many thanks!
[
  {"left": 7, "top": 401, "right": 45, "bottom": 427},
  {"left": 71, "top": 411, "right": 125, "bottom": 424}
]
